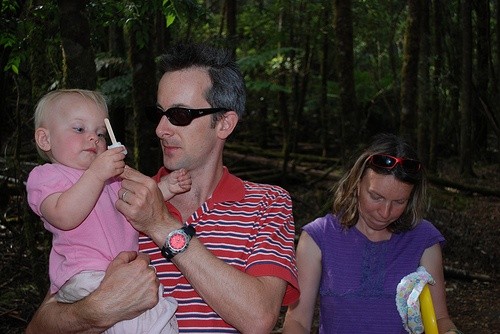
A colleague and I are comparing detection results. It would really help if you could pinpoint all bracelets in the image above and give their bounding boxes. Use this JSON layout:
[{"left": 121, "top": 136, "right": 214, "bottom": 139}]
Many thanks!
[{"left": 444, "top": 328, "right": 461, "bottom": 334}]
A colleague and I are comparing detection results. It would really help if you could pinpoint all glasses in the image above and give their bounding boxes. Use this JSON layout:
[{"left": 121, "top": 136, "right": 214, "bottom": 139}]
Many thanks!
[
  {"left": 156, "top": 106, "right": 228, "bottom": 126},
  {"left": 371, "top": 153, "right": 421, "bottom": 175}
]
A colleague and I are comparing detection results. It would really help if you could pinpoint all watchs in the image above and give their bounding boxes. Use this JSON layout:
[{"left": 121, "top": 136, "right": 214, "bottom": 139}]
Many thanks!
[{"left": 161, "top": 224, "right": 197, "bottom": 262}]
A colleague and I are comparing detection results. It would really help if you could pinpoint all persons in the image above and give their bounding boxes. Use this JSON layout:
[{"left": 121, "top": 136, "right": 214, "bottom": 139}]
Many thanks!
[
  {"left": 27, "top": 39, "right": 302, "bottom": 333},
  {"left": 281, "top": 132, "right": 462, "bottom": 334},
  {"left": 27, "top": 89, "right": 192, "bottom": 334}
]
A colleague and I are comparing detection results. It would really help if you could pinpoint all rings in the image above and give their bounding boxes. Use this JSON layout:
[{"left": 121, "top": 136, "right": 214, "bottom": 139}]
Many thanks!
[
  {"left": 149, "top": 265, "right": 157, "bottom": 274},
  {"left": 122, "top": 190, "right": 130, "bottom": 201}
]
